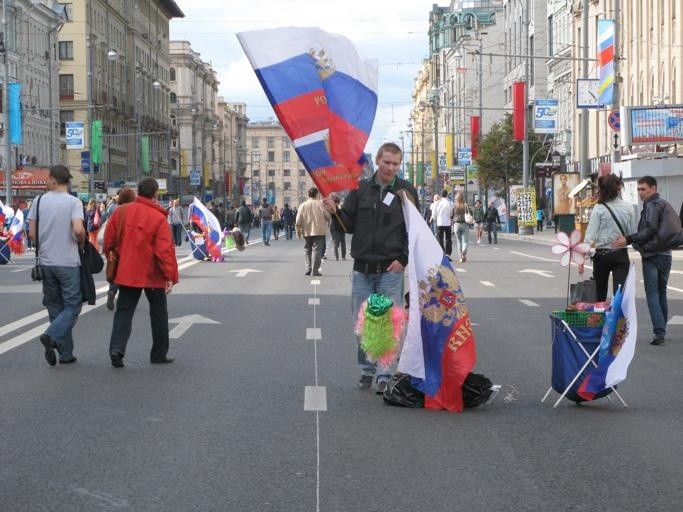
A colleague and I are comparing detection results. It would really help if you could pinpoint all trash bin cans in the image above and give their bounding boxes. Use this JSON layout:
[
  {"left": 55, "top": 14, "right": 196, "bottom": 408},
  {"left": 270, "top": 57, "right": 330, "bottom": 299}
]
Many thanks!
[{"left": 509, "top": 215, "right": 517, "bottom": 233}]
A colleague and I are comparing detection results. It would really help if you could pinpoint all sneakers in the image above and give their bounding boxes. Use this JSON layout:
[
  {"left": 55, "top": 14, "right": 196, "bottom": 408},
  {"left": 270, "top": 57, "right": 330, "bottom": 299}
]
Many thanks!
[
  {"left": 164, "top": 356, "right": 173, "bottom": 364},
  {"left": 106, "top": 246, "right": 120, "bottom": 282},
  {"left": 375, "top": 381, "right": 386, "bottom": 394},
  {"left": 106, "top": 290, "right": 114, "bottom": 311},
  {"left": 650, "top": 337, "right": 663, "bottom": 344},
  {"left": 459, "top": 252, "right": 466, "bottom": 262},
  {"left": 40, "top": 333, "right": 56, "bottom": 366},
  {"left": 111, "top": 351, "right": 124, "bottom": 367},
  {"left": 358, "top": 374, "right": 372, "bottom": 390}
]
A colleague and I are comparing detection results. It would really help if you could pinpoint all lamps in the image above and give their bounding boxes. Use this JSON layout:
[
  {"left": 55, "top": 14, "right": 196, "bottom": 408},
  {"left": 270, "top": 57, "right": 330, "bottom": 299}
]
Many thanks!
[
  {"left": 399, "top": 101, "right": 517, "bottom": 139},
  {"left": 152, "top": 74, "right": 160, "bottom": 89},
  {"left": 93, "top": 42, "right": 117, "bottom": 60},
  {"left": 453, "top": 47, "right": 627, "bottom": 61}
]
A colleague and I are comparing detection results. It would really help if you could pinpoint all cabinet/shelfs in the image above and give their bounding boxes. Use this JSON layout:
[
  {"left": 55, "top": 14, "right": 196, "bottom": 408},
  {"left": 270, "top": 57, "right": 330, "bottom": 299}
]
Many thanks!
[{"left": 574, "top": 197, "right": 594, "bottom": 243}]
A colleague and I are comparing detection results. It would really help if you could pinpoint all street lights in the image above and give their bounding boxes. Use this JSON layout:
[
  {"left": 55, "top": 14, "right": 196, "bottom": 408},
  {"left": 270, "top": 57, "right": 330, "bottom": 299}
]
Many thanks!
[
  {"left": 453, "top": 45, "right": 482, "bottom": 210},
  {"left": 420, "top": 101, "right": 440, "bottom": 195},
  {"left": 134, "top": 76, "right": 270, "bottom": 204},
  {"left": 85, "top": 41, "right": 117, "bottom": 207},
  {"left": 407, "top": 114, "right": 425, "bottom": 194},
  {"left": 400, "top": 130, "right": 415, "bottom": 186}
]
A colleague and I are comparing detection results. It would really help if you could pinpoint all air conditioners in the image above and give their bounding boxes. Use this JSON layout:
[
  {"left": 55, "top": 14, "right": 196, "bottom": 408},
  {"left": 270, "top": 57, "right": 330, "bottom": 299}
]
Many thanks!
[{"left": 96, "top": 193, "right": 106, "bottom": 201}]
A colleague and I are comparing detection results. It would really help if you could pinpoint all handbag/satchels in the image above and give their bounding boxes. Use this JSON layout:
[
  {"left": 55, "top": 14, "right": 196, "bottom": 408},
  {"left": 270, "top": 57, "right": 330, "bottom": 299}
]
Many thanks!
[
  {"left": 31, "top": 266, "right": 42, "bottom": 281},
  {"left": 464, "top": 212, "right": 473, "bottom": 225},
  {"left": 570, "top": 278, "right": 597, "bottom": 304}
]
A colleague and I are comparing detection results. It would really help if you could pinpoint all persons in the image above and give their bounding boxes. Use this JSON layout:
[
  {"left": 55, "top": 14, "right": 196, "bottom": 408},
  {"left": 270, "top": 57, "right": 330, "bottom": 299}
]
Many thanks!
[
  {"left": 610, "top": 174, "right": 683, "bottom": 345},
  {"left": 84, "top": 195, "right": 120, "bottom": 246},
  {"left": 555, "top": 174, "right": 573, "bottom": 214},
  {"left": 21, "top": 200, "right": 36, "bottom": 250},
  {"left": 166, "top": 187, "right": 346, "bottom": 277},
  {"left": 103, "top": 177, "right": 179, "bottom": 367},
  {"left": 27, "top": 165, "right": 86, "bottom": 365},
  {"left": 321, "top": 142, "right": 420, "bottom": 395},
  {"left": 100, "top": 187, "right": 135, "bottom": 310},
  {"left": 578, "top": 173, "right": 636, "bottom": 302},
  {"left": 424, "top": 189, "right": 500, "bottom": 263}
]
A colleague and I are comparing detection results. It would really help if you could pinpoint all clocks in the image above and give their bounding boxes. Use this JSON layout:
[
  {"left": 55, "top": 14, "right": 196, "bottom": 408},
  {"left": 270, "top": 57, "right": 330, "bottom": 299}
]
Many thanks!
[{"left": 577, "top": 79, "right": 604, "bottom": 108}]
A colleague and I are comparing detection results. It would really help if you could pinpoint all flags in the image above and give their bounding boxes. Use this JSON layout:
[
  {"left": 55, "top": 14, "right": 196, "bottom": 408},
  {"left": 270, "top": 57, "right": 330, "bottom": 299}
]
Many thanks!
[
  {"left": 397, "top": 188, "right": 476, "bottom": 413},
  {"left": 577, "top": 264, "right": 638, "bottom": 403},
  {"left": 0, "top": 198, "right": 25, "bottom": 256},
  {"left": 236, "top": 27, "right": 380, "bottom": 198}
]
